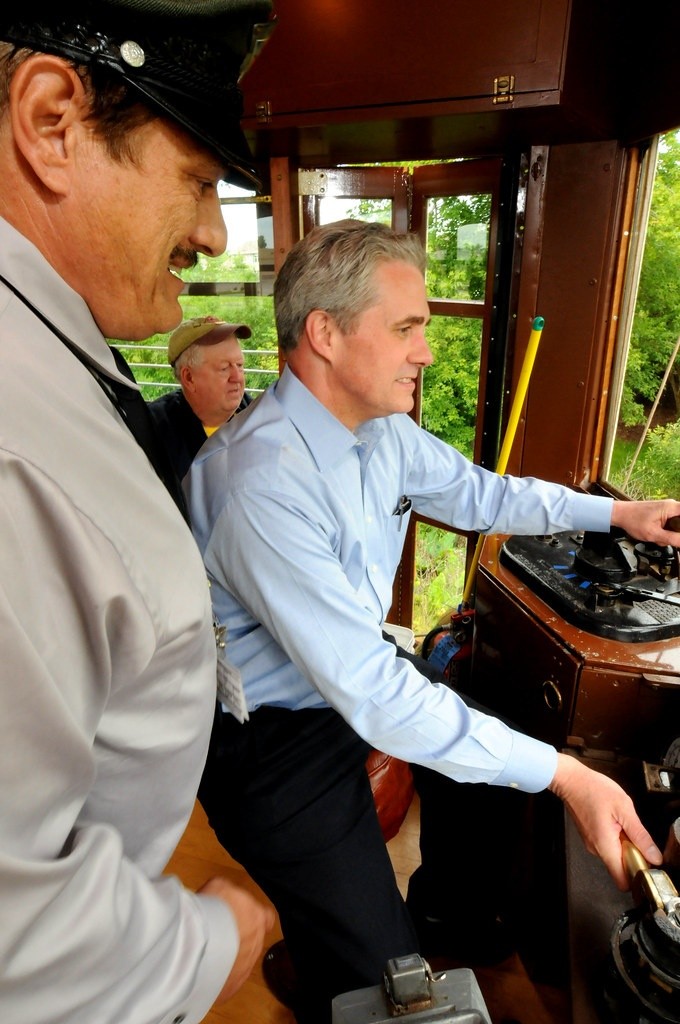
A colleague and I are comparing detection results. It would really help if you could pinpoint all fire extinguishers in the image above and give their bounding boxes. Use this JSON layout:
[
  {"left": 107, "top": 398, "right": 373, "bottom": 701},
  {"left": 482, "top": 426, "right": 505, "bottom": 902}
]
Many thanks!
[{"left": 422, "top": 608, "right": 475, "bottom": 685}]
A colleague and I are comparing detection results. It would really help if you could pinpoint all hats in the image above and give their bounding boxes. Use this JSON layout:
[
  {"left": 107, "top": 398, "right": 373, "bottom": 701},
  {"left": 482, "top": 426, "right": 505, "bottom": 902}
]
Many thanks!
[
  {"left": 167, "top": 318, "right": 252, "bottom": 367},
  {"left": 0, "top": 0, "right": 279, "bottom": 193}
]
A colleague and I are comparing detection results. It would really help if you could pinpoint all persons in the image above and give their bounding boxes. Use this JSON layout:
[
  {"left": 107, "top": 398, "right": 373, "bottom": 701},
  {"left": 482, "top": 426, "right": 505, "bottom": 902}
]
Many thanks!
[
  {"left": 153, "top": 318, "right": 254, "bottom": 480},
  {"left": 0, "top": 2, "right": 276, "bottom": 1024},
  {"left": 189, "top": 220, "right": 679, "bottom": 1024}
]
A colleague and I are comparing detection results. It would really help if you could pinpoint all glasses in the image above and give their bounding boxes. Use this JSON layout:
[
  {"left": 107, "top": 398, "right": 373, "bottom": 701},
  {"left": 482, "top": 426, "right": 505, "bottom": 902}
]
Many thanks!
[{"left": 392, "top": 492, "right": 413, "bottom": 515}]
